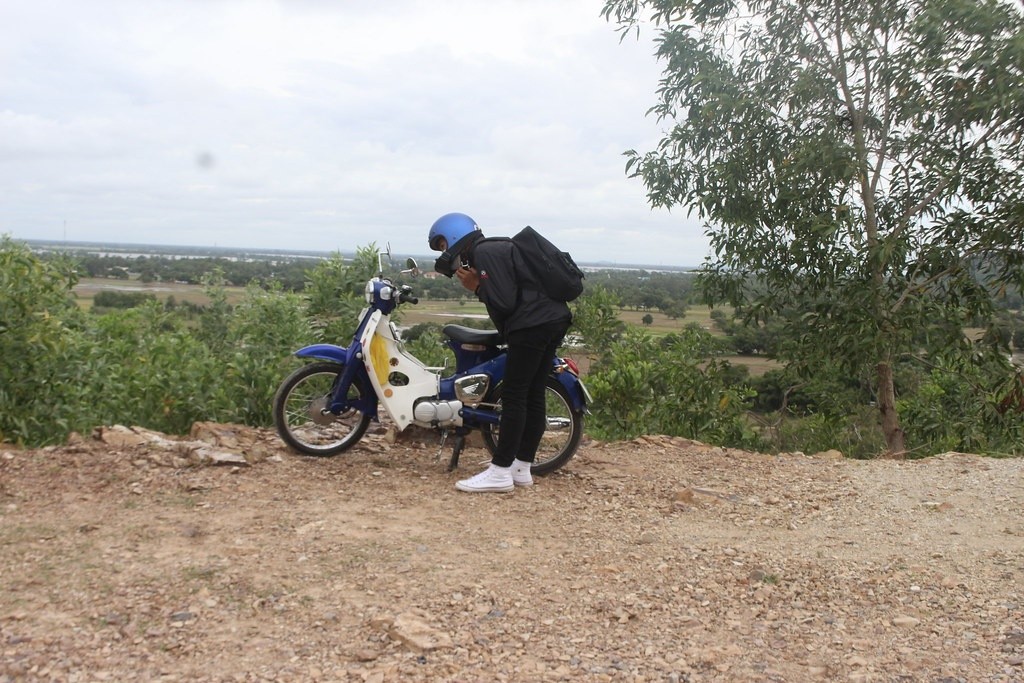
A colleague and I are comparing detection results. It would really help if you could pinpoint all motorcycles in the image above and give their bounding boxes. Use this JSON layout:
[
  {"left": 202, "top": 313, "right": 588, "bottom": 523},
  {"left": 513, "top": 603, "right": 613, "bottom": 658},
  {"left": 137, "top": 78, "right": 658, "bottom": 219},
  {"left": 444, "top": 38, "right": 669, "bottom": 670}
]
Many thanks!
[{"left": 271, "top": 241, "right": 594, "bottom": 478}]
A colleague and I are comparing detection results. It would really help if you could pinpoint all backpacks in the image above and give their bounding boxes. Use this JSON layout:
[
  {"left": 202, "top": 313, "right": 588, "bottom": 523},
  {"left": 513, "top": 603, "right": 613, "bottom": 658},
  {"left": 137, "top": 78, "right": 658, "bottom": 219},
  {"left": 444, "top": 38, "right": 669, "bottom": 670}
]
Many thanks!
[{"left": 468, "top": 226, "right": 586, "bottom": 302}]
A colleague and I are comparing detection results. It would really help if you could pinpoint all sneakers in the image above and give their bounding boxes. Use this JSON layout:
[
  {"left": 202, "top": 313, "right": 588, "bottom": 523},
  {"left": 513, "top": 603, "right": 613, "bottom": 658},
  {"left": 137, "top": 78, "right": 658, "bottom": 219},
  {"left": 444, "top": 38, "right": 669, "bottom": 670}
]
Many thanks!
[
  {"left": 455, "top": 463, "right": 515, "bottom": 492},
  {"left": 510, "top": 461, "right": 533, "bottom": 486}
]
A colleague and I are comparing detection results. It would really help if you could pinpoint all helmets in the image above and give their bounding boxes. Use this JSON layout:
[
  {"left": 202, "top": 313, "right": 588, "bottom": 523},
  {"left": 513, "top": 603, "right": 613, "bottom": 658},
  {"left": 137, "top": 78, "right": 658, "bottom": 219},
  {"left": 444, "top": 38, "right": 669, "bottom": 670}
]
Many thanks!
[{"left": 428, "top": 213, "right": 481, "bottom": 279}]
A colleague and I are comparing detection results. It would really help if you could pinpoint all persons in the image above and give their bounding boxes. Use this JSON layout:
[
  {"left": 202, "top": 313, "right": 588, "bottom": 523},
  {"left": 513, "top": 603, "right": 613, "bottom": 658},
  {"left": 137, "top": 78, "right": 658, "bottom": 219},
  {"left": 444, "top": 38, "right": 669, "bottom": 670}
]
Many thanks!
[{"left": 429, "top": 213, "right": 573, "bottom": 492}]
